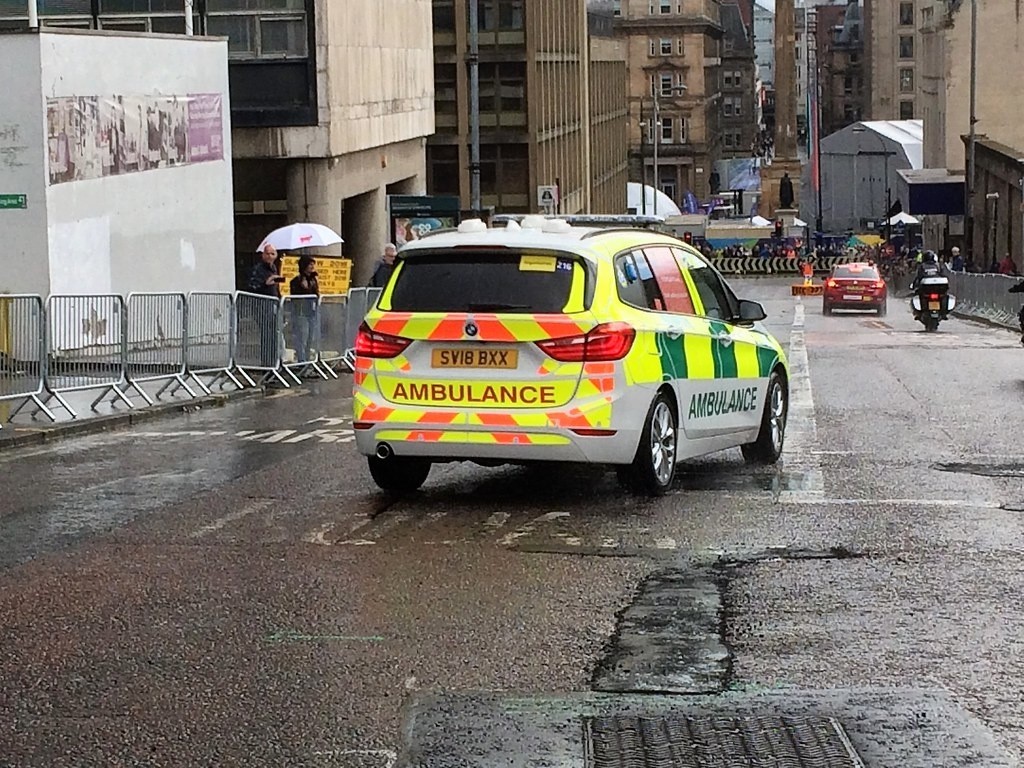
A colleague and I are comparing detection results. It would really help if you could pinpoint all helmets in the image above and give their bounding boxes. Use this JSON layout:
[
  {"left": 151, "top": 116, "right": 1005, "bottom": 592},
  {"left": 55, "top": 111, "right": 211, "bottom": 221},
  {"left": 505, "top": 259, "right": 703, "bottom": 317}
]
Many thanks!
[{"left": 922, "top": 250, "right": 935, "bottom": 261}]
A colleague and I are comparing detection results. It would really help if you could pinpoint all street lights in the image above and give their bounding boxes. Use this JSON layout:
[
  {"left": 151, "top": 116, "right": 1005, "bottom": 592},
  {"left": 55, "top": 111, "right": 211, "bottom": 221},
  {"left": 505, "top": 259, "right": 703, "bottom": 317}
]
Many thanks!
[
  {"left": 653, "top": 85, "right": 688, "bottom": 215},
  {"left": 852, "top": 127, "right": 890, "bottom": 246},
  {"left": 986, "top": 192, "right": 1000, "bottom": 264}
]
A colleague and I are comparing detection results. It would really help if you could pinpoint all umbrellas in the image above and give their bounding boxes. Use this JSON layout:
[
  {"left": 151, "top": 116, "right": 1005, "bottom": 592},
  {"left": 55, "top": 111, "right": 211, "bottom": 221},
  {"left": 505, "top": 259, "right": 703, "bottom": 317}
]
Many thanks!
[
  {"left": 749, "top": 215, "right": 771, "bottom": 226},
  {"left": 794, "top": 218, "right": 807, "bottom": 226},
  {"left": 881, "top": 211, "right": 919, "bottom": 225},
  {"left": 256, "top": 223, "right": 344, "bottom": 281}
]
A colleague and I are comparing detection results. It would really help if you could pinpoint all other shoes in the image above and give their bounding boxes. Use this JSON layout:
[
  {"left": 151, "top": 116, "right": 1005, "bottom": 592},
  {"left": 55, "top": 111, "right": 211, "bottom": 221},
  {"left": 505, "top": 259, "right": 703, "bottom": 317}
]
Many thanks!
[
  {"left": 300, "top": 363, "right": 321, "bottom": 377},
  {"left": 264, "top": 369, "right": 279, "bottom": 384}
]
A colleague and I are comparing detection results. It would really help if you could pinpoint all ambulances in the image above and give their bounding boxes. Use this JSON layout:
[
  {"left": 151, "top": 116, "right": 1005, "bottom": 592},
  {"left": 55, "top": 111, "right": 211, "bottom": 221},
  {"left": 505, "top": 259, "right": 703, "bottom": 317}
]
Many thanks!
[{"left": 350, "top": 215, "right": 789, "bottom": 497}]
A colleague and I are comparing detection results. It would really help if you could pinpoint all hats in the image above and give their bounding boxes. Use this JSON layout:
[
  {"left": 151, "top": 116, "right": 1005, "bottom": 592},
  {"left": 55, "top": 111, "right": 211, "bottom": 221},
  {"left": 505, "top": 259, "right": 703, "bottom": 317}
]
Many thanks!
[
  {"left": 298, "top": 253, "right": 315, "bottom": 273},
  {"left": 951, "top": 246, "right": 959, "bottom": 252}
]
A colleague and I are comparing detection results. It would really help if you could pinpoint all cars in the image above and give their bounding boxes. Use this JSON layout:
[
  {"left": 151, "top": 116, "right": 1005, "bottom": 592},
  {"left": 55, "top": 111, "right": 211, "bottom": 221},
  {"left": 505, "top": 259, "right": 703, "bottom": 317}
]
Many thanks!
[{"left": 822, "top": 263, "right": 887, "bottom": 316}]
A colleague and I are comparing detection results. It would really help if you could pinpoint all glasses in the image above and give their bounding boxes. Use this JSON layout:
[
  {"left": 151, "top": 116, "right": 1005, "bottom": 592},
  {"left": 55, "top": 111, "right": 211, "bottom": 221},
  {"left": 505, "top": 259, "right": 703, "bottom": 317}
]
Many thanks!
[{"left": 266, "top": 251, "right": 275, "bottom": 256}]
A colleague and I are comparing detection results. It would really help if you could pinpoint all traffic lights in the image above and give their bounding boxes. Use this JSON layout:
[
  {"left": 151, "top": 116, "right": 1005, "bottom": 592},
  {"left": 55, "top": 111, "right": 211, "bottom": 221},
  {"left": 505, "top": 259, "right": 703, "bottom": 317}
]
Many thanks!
[
  {"left": 684, "top": 231, "right": 692, "bottom": 244},
  {"left": 775, "top": 221, "right": 782, "bottom": 237}
]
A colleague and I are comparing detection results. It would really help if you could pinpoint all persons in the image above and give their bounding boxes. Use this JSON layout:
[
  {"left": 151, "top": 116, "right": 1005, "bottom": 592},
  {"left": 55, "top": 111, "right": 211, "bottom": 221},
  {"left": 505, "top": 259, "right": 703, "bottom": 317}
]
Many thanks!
[
  {"left": 1000, "top": 252, "right": 1014, "bottom": 275},
  {"left": 692, "top": 238, "right": 948, "bottom": 277},
  {"left": 249, "top": 244, "right": 284, "bottom": 383},
  {"left": 289, "top": 256, "right": 323, "bottom": 378},
  {"left": 47, "top": 95, "right": 189, "bottom": 184},
  {"left": 913, "top": 250, "right": 949, "bottom": 320},
  {"left": 365, "top": 243, "right": 401, "bottom": 289},
  {"left": 951, "top": 247, "right": 963, "bottom": 272}
]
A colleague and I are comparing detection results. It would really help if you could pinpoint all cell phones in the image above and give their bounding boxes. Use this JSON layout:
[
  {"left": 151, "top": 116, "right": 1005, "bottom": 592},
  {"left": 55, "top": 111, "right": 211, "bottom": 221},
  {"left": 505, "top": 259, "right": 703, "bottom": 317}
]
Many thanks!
[{"left": 274, "top": 277, "right": 285, "bottom": 283}]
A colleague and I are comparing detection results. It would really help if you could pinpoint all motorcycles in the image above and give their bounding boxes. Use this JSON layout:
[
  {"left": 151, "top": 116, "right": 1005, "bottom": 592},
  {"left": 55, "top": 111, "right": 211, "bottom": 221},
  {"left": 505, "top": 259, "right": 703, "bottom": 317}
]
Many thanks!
[{"left": 910, "top": 277, "right": 949, "bottom": 333}]
